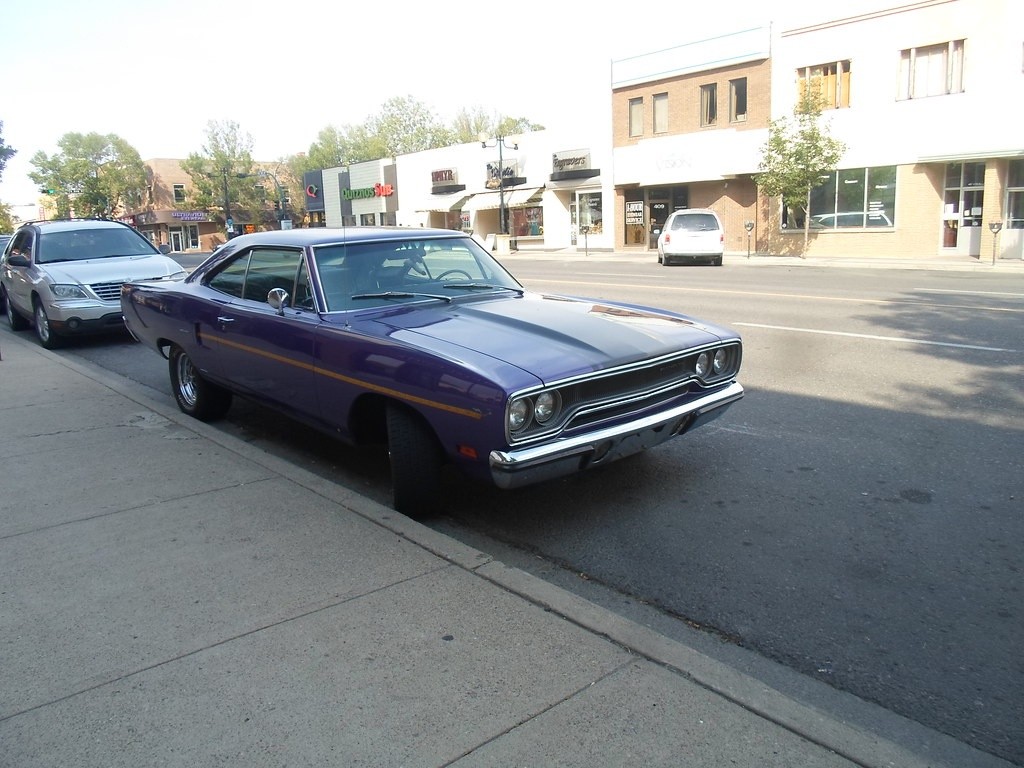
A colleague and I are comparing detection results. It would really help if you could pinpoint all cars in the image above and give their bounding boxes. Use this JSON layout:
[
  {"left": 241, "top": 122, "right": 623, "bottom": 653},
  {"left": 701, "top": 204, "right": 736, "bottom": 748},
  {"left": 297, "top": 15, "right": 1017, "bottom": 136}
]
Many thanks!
[
  {"left": 0, "top": 234, "right": 13, "bottom": 263},
  {"left": 0, "top": 217, "right": 189, "bottom": 350},
  {"left": 119, "top": 226, "right": 747, "bottom": 523},
  {"left": 652, "top": 208, "right": 726, "bottom": 266}
]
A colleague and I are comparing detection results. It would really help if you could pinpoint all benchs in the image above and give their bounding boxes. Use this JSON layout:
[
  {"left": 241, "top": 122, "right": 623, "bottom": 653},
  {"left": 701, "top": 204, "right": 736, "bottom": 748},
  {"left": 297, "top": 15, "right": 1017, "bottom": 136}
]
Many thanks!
[{"left": 212, "top": 266, "right": 363, "bottom": 304}]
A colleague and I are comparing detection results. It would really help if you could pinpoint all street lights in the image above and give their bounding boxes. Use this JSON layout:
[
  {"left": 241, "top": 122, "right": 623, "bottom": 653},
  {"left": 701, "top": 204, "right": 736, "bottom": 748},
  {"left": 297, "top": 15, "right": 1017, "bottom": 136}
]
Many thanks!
[
  {"left": 207, "top": 167, "right": 246, "bottom": 241},
  {"left": 477, "top": 127, "right": 522, "bottom": 236}
]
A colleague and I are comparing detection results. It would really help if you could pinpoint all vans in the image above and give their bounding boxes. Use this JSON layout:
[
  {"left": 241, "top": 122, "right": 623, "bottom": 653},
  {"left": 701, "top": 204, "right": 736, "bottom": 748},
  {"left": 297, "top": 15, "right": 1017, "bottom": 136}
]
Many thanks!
[{"left": 810, "top": 211, "right": 892, "bottom": 228}]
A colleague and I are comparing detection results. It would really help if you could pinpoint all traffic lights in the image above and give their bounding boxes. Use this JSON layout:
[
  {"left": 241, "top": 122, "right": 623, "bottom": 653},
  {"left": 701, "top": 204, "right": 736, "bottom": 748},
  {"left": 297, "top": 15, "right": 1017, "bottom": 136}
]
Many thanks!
[
  {"left": 275, "top": 199, "right": 279, "bottom": 210},
  {"left": 46, "top": 188, "right": 56, "bottom": 194}
]
